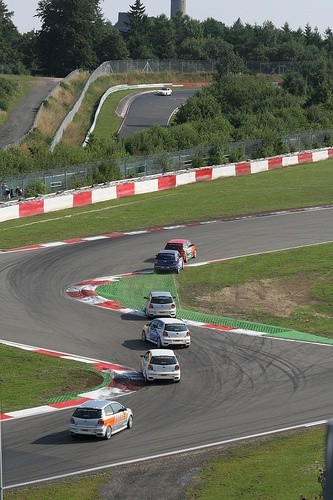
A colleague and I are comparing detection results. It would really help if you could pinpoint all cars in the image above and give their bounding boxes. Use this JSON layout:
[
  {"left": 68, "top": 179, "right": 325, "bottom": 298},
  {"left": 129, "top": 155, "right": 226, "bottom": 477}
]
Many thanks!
[
  {"left": 143, "top": 291, "right": 177, "bottom": 320},
  {"left": 164, "top": 238, "right": 197, "bottom": 263},
  {"left": 69, "top": 399, "right": 133, "bottom": 440},
  {"left": 154, "top": 249, "right": 184, "bottom": 274},
  {"left": 141, "top": 318, "right": 191, "bottom": 348},
  {"left": 158, "top": 87, "right": 173, "bottom": 96},
  {"left": 139, "top": 348, "right": 181, "bottom": 384}
]
